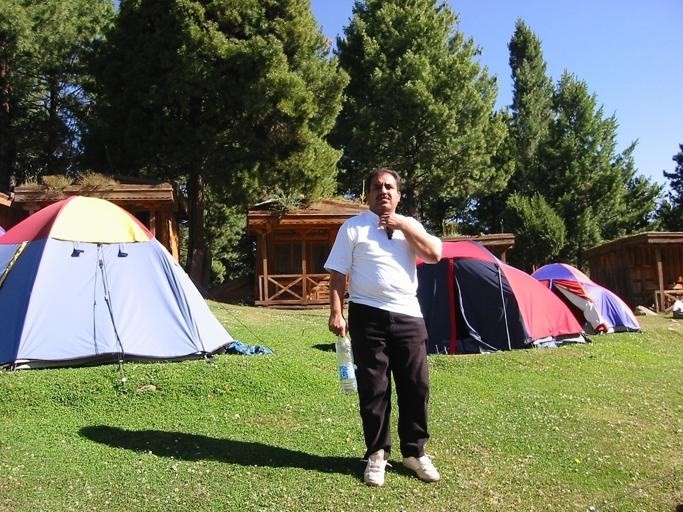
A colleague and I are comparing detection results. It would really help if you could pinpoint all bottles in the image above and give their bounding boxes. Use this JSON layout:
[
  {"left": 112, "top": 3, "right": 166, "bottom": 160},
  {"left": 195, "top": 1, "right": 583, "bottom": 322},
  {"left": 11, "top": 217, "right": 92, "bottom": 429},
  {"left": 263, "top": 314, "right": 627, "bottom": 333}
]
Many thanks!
[{"left": 336, "top": 334, "right": 358, "bottom": 395}]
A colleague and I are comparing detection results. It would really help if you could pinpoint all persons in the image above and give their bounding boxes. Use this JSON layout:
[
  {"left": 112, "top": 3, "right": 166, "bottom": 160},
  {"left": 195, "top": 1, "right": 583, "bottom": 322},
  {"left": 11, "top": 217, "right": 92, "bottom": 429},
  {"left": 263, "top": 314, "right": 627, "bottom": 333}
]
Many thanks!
[{"left": 321, "top": 167, "right": 444, "bottom": 488}]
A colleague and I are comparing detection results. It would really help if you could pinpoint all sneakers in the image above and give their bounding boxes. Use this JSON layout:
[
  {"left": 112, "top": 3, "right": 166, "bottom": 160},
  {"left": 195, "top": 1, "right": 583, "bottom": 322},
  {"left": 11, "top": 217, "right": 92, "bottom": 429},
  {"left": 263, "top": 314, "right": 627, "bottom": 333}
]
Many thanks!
[
  {"left": 402, "top": 454, "right": 440, "bottom": 483},
  {"left": 364, "top": 448, "right": 387, "bottom": 486}
]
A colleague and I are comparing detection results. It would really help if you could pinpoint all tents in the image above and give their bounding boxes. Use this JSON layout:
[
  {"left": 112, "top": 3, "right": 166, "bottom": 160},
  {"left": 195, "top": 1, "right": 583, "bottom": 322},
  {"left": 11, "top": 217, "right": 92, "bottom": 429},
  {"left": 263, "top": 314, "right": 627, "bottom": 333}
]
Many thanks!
[
  {"left": 412, "top": 238, "right": 592, "bottom": 355},
  {"left": 529, "top": 262, "right": 641, "bottom": 337},
  {"left": 0, "top": 194, "right": 272, "bottom": 372}
]
